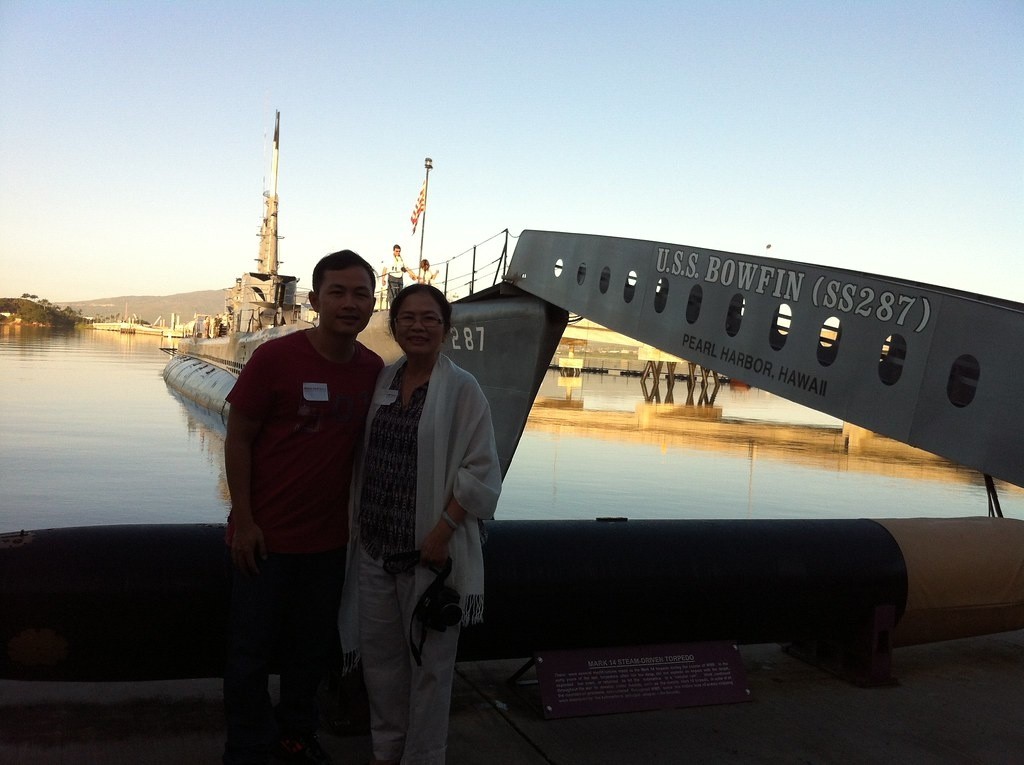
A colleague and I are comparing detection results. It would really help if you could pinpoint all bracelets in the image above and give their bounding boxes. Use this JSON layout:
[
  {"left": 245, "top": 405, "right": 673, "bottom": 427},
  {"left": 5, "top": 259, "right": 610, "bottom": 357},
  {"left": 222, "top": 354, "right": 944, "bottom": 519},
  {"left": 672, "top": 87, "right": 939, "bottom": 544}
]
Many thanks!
[{"left": 441, "top": 510, "right": 459, "bottom": 531}]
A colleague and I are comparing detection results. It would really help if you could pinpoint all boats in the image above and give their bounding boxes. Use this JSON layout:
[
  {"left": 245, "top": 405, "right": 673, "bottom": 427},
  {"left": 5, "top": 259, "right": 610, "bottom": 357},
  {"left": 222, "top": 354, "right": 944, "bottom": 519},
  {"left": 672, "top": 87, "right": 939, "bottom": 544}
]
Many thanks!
[{"left": 161, "top": 104, "right": 1024, "bottom": 519}]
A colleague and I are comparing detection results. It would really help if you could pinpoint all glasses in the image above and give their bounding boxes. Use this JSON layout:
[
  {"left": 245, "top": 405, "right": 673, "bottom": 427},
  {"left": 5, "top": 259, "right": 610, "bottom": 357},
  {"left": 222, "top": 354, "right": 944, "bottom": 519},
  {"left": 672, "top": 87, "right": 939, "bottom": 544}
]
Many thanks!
[{"left": 395, "top": 314, "right": 443, "bottom": 328}]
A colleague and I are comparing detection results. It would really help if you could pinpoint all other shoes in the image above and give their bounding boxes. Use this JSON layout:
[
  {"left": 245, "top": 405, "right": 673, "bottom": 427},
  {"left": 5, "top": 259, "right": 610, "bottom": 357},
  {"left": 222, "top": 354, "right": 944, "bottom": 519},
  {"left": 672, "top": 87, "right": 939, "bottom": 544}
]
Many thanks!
[
  {"left": 273, "top": 725, "right": 334, "bottom": 765},
  {"left": 221, "top": 742, "right": 268, "bottom": 765}
]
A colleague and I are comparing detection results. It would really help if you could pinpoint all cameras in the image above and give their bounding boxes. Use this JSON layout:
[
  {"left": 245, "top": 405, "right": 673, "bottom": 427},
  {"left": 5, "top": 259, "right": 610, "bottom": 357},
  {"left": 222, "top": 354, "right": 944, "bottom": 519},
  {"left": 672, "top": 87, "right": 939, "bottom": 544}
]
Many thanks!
[{"left": 416, "top": 584, "right": 462, "bottom": 632}]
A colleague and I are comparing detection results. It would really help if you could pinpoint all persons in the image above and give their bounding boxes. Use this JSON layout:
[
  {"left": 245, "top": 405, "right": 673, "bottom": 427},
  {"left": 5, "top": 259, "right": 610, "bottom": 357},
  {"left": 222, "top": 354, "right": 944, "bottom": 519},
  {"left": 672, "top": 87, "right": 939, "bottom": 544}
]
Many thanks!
[
  {"left": 382, "top": 244, "right": 417, "bottom": 308},
  {"left": 204, "top": 313, "right": 222, "bottom": 338},
  {"left": 224, "top": 249, "right": 385, "bottom": 765},
  {"left": 413, "top": 260, "right": 440, "bottom": 286},
  {"left": 339, "top": 282, "right": 502, "bottom": 765}
]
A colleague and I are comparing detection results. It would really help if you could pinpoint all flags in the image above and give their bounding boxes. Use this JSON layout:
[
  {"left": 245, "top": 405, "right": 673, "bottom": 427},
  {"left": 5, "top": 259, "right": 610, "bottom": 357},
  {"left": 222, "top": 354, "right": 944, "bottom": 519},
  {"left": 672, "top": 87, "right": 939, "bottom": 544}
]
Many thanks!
[{"left": 410, "top": 180, "right": 426, "bottom": 235}]
